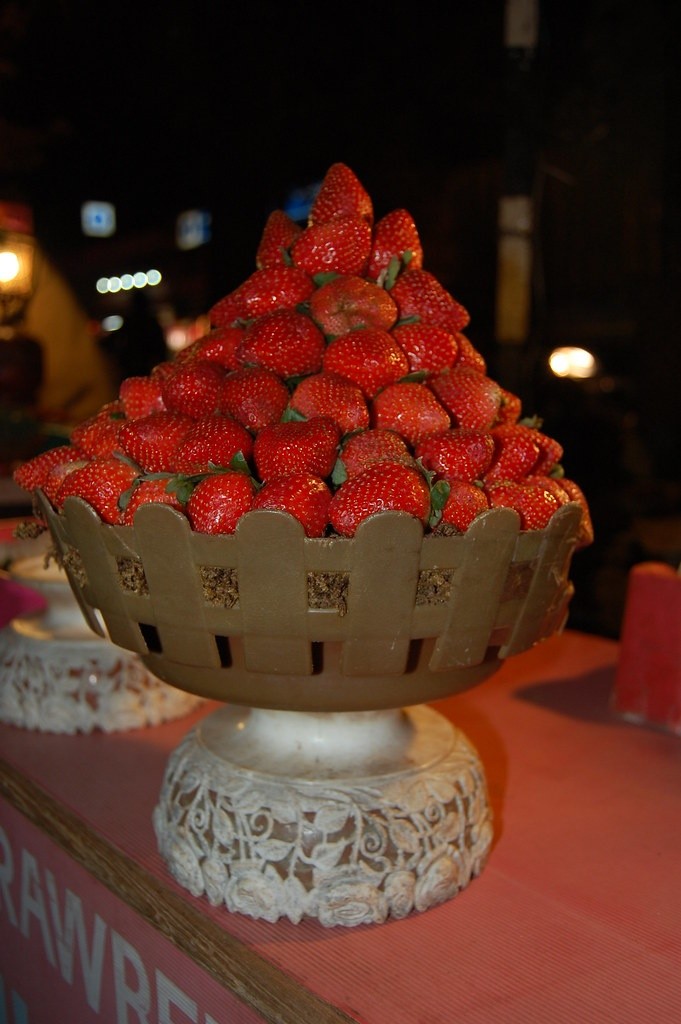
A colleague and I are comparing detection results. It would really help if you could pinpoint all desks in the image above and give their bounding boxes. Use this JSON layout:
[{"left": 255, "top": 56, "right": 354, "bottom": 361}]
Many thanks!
[{"left": 0, "top": 505, "right": 681, "bottom": 1024}]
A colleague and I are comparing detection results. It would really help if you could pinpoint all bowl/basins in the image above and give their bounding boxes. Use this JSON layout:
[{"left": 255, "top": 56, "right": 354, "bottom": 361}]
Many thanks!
[{"left": 10, "top": 556, "right": 106, "bottom": 637}]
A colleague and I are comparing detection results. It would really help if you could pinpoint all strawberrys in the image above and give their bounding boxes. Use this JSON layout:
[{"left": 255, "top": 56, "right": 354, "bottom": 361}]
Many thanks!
[{"left": 12, "top": 163, "right": 594, "bottom": 546}]
